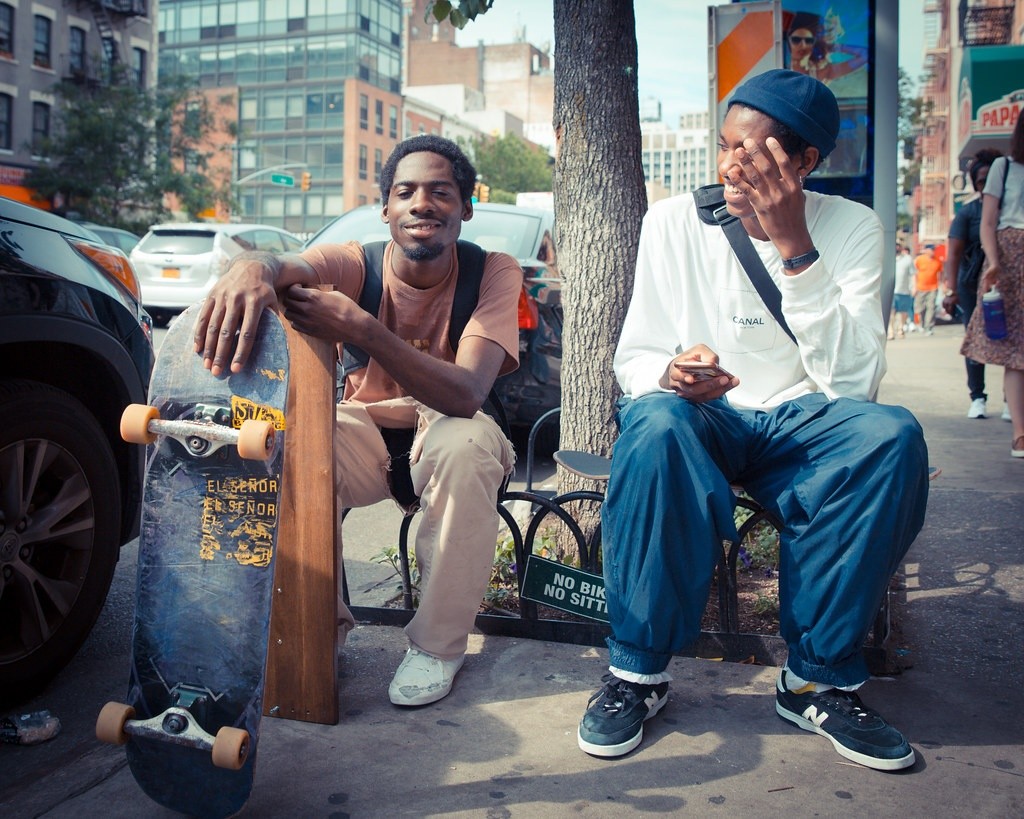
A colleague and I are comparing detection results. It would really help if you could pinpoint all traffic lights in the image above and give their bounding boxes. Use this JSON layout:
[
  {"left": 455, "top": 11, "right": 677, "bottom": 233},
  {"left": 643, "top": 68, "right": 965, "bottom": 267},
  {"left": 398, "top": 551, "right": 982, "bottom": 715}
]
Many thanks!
[{"left": 300, "top": 171, "right": 312, "bottom": 192}]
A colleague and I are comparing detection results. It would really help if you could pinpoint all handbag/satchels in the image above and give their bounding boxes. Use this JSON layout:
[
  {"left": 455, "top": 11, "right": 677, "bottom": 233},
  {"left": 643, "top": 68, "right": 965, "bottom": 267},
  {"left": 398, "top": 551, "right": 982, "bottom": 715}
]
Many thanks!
[{"left": 960, "top": 241, "right": 985, "bottom": 285}]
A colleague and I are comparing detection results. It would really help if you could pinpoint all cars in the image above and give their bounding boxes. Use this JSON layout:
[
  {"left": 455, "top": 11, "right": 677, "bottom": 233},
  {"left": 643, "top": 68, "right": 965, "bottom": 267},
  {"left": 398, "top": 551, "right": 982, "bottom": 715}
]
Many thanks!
[
  {"left": 129, "top": 221, "right": 305, "bottom": 328},
  {"left": 56, "top": 211, "right": 141, "bottom": 260},
  {"left": 290, "top": 197, "right": 562, "bottom": 467}
]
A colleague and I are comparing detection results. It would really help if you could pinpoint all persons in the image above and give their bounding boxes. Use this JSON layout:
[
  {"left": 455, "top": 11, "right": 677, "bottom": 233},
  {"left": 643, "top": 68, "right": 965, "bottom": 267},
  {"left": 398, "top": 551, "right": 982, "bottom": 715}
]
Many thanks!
[
  {"left": 578, "top": 67, "right": 929, "bottom": 774},
  {"left": 959, "top": 108, "right": 1024, "bottom": 460},
  {"left": 194, "top": 133, "right": 527, "bottom": 708},
  {"left": 887, "top": 235, "right": 947, "bottom": 341},
  {"left": 941, "top": 148, "right": 1015, "bottom": 422},
  {"left": 780, "top": 21, "right": 833, "bottom": 87}
]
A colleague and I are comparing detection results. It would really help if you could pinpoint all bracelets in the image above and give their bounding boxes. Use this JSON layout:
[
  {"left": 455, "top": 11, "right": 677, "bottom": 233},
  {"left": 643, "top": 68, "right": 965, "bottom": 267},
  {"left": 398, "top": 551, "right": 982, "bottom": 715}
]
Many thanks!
[{"left": 782, "top": 250, "right": 819, "bottom": 271}]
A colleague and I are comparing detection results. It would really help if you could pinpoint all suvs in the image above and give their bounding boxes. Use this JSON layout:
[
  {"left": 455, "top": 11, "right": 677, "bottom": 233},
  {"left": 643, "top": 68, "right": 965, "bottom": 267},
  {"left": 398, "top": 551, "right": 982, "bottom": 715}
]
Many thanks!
[{"left": 0, "top": 192, "right": 156, "bottom": 707}]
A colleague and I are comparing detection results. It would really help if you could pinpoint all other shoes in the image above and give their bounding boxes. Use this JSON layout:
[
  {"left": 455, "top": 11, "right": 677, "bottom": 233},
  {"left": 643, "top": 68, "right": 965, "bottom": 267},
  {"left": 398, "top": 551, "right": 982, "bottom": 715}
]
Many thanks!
[
  {"left": 887, "top": 322, "right": 933, "bottom": 340},
  {"left": 1011, "top": 436, "right": 1024, "bottom": 457}
]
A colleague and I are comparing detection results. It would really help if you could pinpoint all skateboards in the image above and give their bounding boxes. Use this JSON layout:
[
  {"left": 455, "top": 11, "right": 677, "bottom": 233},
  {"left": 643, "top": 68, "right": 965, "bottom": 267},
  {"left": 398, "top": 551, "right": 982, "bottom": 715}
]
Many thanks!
[
  {"left": 95, "top": 297, "right": 291, "bottom": 819},
  {"left": 552, "top": 448, "right": 612, "bottom": 481}
]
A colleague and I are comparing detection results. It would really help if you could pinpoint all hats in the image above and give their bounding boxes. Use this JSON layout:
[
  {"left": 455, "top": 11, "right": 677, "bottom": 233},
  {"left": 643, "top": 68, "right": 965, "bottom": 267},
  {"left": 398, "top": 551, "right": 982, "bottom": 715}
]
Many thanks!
[
  {"left": 728, "top": 69, "right": 841, "bottom": 158},
  {"left": 926, "top": 244, "right": 935, "bottom": 248}
]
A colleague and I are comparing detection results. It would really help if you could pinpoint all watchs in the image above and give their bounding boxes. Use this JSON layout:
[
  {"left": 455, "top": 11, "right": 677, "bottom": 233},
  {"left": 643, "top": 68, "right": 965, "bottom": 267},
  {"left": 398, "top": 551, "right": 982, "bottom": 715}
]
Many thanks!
[{"left": 944, "top": 288, "right": 958, "bottom": 297}]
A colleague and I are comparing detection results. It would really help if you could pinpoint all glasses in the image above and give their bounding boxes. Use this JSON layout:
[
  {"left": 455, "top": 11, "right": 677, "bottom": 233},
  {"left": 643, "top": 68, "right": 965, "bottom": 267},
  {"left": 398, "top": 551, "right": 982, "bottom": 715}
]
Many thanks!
[{"left": 791, "top": 37, "right": 816, "bottom": 44}]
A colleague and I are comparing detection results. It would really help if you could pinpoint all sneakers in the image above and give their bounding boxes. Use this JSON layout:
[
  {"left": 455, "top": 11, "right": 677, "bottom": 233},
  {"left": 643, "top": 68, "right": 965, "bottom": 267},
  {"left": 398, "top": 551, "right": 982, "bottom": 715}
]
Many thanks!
[
  {"left": 1001, "top": 405, "right": 1012, "bottom": 421},
  {"left": 388, "top": 648, "right": 464, "bottom": 705},
  {"left": 968, "top": 398, "right": 988, "bottom": 419},
  {"left": 577, "top": 673, "right": 669, "bottom": 756},
  {"left": 776, "top": 667, "right": 915, "bottom": 769}
]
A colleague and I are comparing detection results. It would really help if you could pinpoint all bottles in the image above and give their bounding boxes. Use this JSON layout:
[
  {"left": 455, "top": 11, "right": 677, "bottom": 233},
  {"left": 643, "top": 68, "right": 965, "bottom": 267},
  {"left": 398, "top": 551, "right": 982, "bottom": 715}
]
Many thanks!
[{"left": 981, "top": 284, "right": 1009, "bottom": 341}]
